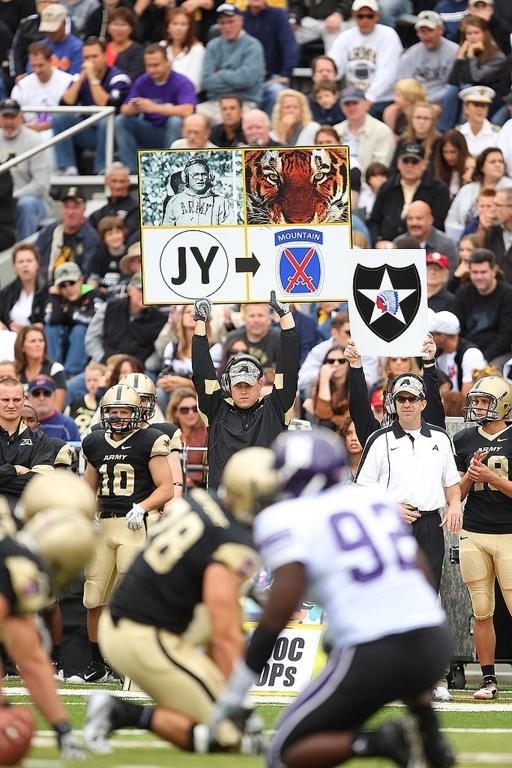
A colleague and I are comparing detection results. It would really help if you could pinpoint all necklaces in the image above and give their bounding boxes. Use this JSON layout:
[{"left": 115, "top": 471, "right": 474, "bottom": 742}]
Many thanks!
[{"left": 21, "top": 382, "right": 56, "bottom": 468}]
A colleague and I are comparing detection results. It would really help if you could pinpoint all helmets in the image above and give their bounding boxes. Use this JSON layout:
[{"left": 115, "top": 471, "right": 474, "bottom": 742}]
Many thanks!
[
  {"left": 14, "top": 469, "right": 98, "bottom": 522},
  {"left": 118, "top": 372, "right": 157, "bottom": 421},
  {"left": 98, "top": 382, "right": 142, "bottom": 434},
  {"left": 15, "top": 504, "right": 97, "bottom": 592},
  {"left": 462, "top": 376, "right": 512, "bottom": 424},
  {"left": 273, "top": 423, "right": 352, "bottom": 492},
  {"left": 218, "top": 444, "right": 282, "bottom": 525}
]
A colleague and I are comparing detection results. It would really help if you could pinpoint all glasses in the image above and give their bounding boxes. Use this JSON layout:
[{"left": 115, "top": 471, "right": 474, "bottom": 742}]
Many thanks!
[
  {"left": 30, "top": 388, "right": 51, "bottom": 397},
  {"left": 353, "top": 12, "right": 377, "bottom": 19},
  {"left": 57, "top": 280, "right": 76, "bottom": 288},
  {"left": 428, "top": 263, "right": 444, "bottom": 272},
  {"left": 339, "top": 328, "right": 351, "bottom": 336},
  {"left": 229, "top": 350, "right": 249, "bottom": 355},
  {"left": 401, "top": 156, "right": 420, "bottom": 164},
  {"left": 179, "top": 405, "right": 198, "bottom": 415},
  {"left": 188, "top": 173, "right": 210, "bottom": 179},
  {"left": 325, "top": 358, "right": 348, "bottom": 364},
  {"left": 396, "top": 395, "right": 419, "bottom": 403}
]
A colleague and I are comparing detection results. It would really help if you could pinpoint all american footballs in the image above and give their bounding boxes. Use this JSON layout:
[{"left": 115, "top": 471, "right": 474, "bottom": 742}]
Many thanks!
[{"left": 2, "top": 706, "right": 36, "bottom": 767}]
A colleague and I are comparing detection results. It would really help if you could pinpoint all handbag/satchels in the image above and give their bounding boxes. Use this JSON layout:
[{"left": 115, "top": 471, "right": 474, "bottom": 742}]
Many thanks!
[{"left": 157, "top": 339, "right": 190, "bottom": 380}]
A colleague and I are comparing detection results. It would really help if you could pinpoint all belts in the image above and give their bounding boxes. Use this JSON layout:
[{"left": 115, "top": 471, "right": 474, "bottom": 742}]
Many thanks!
[{"left": 100, "top": 511, "right": 126, "bottom": 519}]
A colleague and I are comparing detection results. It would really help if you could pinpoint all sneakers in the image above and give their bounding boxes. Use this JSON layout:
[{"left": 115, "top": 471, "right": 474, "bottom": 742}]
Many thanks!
[
  {"left": 474, "top": 678, "right": 499, "bottom": 699},
  {"left": 432, "top": 687, "right": 454, "bottom": 701},
  {"left": 412, "top": 703, "right": 456, "bottom": 767},
  {"left": 375, "top": 717, "right": 420, "bottom": 766},
  {"left": 66, "top": 659, "right": 109, "bottom": 684},
  {"left": 83, "top": 689, "right": 116, "bottom": 758}
]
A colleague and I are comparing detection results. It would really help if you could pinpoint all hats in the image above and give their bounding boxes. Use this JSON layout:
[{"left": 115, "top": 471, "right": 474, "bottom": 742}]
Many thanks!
[
  {"left": 392, "top": 371, "right": 427, "bottom": 400},
  {"left": 415, "top": 9, "right": 444, "bottom": 30},
  {"left": 0, "top": 98, "right": 20, "bottom": 116},
  {"left": 60, "top": 186, "right": 87, "bottom": 202},
  {"left": 216, "top": 2, "right": 242, "bottom": 17},
  {"left": 351, "top": 0, "right": 380, "bottom": 12},
  {"left": 119, "top": 241, "right": 141, "bottom": 275},
  {"left": 458, "top": 85, "right": 497, "bottom": 104},
  {"left": 339, "top": 85, "right": 366, "bottom": 104},
  {"left": 429, "top": 309, "right": 462, "bottom": 335},
  {"left": 229, "top": 354, "right": 263, "bottom": 386},
  {"left": 29, "top": 373, "right": 56, "bottom": 392},
  {"left": 372, "top": 387, "right": 387, "bottom": 408},
  {"left": 469, "top": 0, "right": 495, "bottom": 6},
  {"left": 398, "top": 142, "right": 426, "bottom": 160},
  {"left": 53, "top": 261, "right": 82, "bottom": 286},
  {"left": 129, "top": 271, "right": 142, "bottom": 289},
  {"left": 37, "top": 2, "right": 68, "bottom": 33},
  {"left": 427, "top": 251, "right": 450, "bottom": 268}
]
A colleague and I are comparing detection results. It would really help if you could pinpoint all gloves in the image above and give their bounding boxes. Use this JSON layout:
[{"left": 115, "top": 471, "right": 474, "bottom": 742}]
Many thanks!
[
  {"left": 268, "top": 289, "right": 291, "bottom": 318},
  {"left": 57, "top": 732, "right": 87, "bottom": 763},
  {"left": 204, "top": 662, "right": 257, "bottom": 743},
  {"left": 193, "top": 297, "right": 211, "bottom": 322},
  {"left": 92, "top": 513, "right": 102, "bottom": 533},
  {"left": 125, "top": 502, "right": 146, "bottom": 532}
]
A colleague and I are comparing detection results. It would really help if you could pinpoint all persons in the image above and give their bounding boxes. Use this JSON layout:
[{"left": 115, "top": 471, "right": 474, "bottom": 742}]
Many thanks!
[
  {"left": 1, "top": 4, "right": 512, "bottom": 148},
  {"left": 208, "top": 303, "right": 300, "bottom": 388},
  {"left": 211, "top": 419, "right": 462, "bottom": 768},
  {"left": 163, "top": 156, "right": 236, "bottom": 226},
  {"left": 337, "top": 416, "right": 363, "bottom": 483},
  {"left": 0, "top": 469, "right": 100, "bottom": 548},
  {"left": 0, "top": 376, "right": 56, "bottom": 497},
  {"left": 351, "top": 149, "right": 511, "bottom": 251},
  {"left": 118, "top": 373, "right": 185, "bottom": 541},
  {"left": 300, "top": 300, "right": 349, "bottom": 431},
  {"left": 175, "top": 304, "right": 208, "bottom": 493},
  {"left": 147, "top": 383, "right": 175, "bottom": 426},
  {"left": 70, "top": 382, "right": 176, "bottom": 687},
  {"left": 2, "top": 151, "right": 176, "bottom": 382},
  {"left": 86, "top": 443, "right": 299, "bottom": 758},
  {"left": 451, "top": 374, "right": 511, "bottom": 703},
  {"left": 361, "top": 354, "right": 428, "bottom": 425},
  {"left": 428, "top": 250, "right": 511, "bottom": 416},
  {"left": 1, "top": 505, "right": 98, "bottom": 768},
  {"left": 192, "top": 290, "right": 300, "bottom": 502},
  {"left": 44, "top": 606, "right": 64, "bottom": 682},
  {"left": 55, "top": 382, "right": 118, "bottom": 472},
  {"left": 354, "top": 373, "right": 462, "bottom": 703}
]
